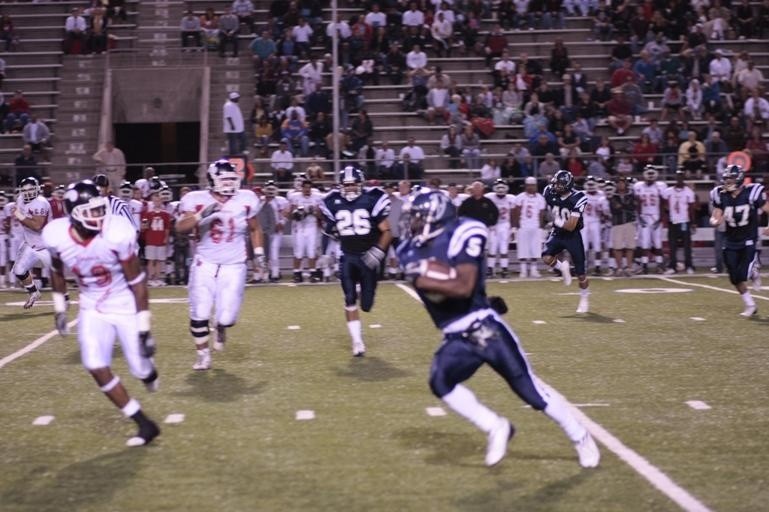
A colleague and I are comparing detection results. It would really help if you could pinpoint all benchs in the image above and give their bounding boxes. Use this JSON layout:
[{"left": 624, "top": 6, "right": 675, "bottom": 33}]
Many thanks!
[{"left": 0, "top": 0, "right": 769, "bottom": 183}]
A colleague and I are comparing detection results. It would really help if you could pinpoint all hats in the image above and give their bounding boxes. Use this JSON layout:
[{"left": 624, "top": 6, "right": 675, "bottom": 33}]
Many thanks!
[{"left": 229, "top": 92, "right": 240, "bottom": 99}]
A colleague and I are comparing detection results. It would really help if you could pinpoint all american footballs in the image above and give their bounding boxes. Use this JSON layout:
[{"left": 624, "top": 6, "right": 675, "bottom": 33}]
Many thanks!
[{"left": 409, "top": 258, "right": 458, "bottom": 281}]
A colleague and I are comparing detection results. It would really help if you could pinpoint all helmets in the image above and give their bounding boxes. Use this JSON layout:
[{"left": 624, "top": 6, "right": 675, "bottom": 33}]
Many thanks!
[
  {"left": 491, "top": 163, "right": 746, "bottom": 195},
  {"left": 0, "top": 176, "right": 172, "bottom": 230},
  {"left": 293, "top": 173, "right": 310, "bottom": 190},
  {"left": 263, "top": 181, "right": 277, "bottom": 198},
  {"left": 338, "top": 166, "right": 364, "bottom": 201},
  {"left": 410, "top": 190, "right": 455, "bottom": 247},
  {"left": 207, "top": 160, "right": 237, "bottom": 196}
]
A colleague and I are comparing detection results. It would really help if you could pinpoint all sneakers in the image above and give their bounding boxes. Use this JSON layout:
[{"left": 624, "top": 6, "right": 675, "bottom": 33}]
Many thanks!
[
  {"left": 739, "top": 261, "right": 761, "bottom": 317},
  {"left": 24, "top": 290, "right": 41, "bottom": 308},
  {"left": 147, "top": 279, "right": 167, "bottom": 288},
  {"left": 128, "top": 422, "right": 160, "bottom": 446},
  {"left": 576, "top": 293, "right": 589, "bottom": 313},
  {"left": 486, "top": 261, "right": 695, "bottom": 286},
  {"left": 353, "top": 337, "right": 366, "bottom": 355},
  {"left": 571, "top": 424, "right": 600, "bottom": 467},
  {"left": 485, "top": 418, "right": 515, "bottom": 466},
  {"left": 191, "top": 328, "right": 225, "bottom": 369},
  {"left": 248, "top": 266, "right": 403, "bottom": 282}
]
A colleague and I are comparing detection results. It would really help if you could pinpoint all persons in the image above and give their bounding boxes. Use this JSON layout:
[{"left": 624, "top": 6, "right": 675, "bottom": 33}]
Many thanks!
[
  {"left": 388, "top": 190, "right": 601, "bottom": 469},
  {"left": 40, "top": 178, "right": 160, "bottom": 448},
  {"left": 0, "top": 0, "right": 769, "bottom": 370}
]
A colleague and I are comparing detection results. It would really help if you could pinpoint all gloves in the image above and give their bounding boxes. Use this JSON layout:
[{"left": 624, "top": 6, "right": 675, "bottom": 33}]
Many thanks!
[
  {"left": 55, "top": 313, "right": 69, "bottom": 336},
  {"left": 196, "top": 201, "right": 222, "bottom": 221},
  {"left": 252, "top": 254, "right": 265, "bottom": 269},
  {"left": 140, "top": 331, "right": 156, "bottom": 357}
]
